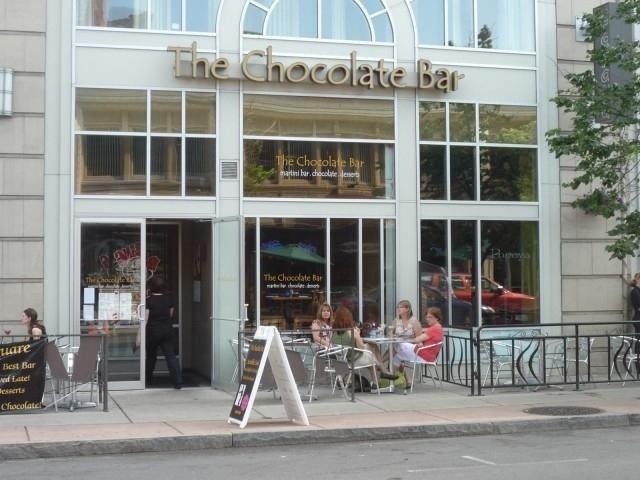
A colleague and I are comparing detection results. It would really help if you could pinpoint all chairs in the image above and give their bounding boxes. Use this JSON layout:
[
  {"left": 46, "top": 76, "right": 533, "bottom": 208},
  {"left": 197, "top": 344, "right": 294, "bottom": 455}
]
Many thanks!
[
  {"left": 481, "top": 336, "right": 525, "bottom": 392},
  {"left": 43, "top": 333, "right": 104, "bottom": 406},
  {"left": 230, "top": 319, "right": 445, "bottom": 402},
  {"left": 546, "top": 336, "right": 597, "bottom": 389},
  {"left": 607, "top": 335, "right": 639, "bottom": 387}
]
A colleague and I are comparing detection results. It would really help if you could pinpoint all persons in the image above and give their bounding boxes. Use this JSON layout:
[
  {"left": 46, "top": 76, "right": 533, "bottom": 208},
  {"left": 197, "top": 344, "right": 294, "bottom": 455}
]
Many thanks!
[
  {"left": 131, "top": 276, "right": 184, "bottom": 390},
  {"left": 627, "top": 272, "right": 640, "bottom": 377},
  {"left": 21, "top": 306, "right": 48, "bottom": 342},
  {"left": 310, "top": 298, "right": 446, "bottom": 394}
]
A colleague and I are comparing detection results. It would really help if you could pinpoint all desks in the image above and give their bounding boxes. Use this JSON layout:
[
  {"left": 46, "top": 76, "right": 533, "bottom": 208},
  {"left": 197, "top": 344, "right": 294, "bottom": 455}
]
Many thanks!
[{"left": 261, "top": 315, "right": 315, "bottom": 329}]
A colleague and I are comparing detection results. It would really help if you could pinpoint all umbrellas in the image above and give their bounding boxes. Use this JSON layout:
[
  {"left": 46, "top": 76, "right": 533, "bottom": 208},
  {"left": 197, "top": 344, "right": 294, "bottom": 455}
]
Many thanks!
[{"left": 248, "top": 242, "right": 335, "bottom": 269}]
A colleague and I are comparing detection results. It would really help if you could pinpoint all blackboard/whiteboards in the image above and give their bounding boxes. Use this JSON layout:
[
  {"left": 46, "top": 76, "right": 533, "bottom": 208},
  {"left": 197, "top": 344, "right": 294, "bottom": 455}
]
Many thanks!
[{"left": 229, "top": 339, "right": 267, "bottom": 421}]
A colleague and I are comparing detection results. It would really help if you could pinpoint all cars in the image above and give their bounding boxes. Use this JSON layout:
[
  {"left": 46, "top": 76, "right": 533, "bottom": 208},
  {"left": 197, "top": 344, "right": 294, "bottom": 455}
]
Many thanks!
[{"left": 328, "top": 265, "right": 539, "bottom": 330}]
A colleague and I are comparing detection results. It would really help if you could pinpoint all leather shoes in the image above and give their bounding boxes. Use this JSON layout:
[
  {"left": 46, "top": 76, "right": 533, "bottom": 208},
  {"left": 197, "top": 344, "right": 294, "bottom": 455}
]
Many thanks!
[
  {"left": 380, "top": 372, "right": 399, "bottom": 380},
  {"left": 406, "top": 382, "right": 414, "bottom": 389}
]
